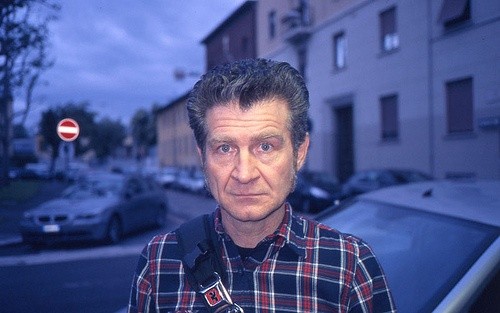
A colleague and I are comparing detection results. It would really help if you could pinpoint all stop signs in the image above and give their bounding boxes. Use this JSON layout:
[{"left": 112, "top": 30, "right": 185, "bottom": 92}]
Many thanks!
[{"left": 56, "top": 117, "right": 80, "bottom": 142}]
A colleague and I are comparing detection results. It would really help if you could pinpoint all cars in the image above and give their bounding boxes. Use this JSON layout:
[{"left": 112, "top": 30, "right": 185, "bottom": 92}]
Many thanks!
[
  {"left": 21, "top": 172, "right": 169, "bottom": 249},
  {"left": 112, "top": 159, "right": 436, "bottom": 214},
  {"left": 309, "top": 175, "right": 500, "bottom": 313}
]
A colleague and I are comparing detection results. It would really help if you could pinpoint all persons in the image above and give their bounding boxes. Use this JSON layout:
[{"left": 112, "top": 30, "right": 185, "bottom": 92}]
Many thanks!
[{"left": 125, "top": 57, "right": 400, "bottom": 313}]
